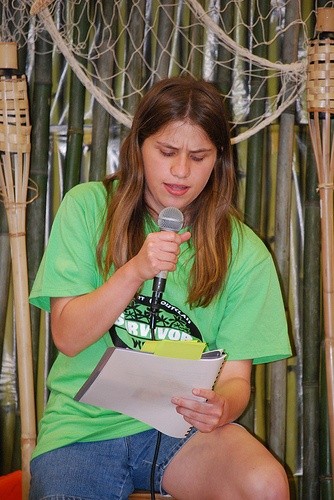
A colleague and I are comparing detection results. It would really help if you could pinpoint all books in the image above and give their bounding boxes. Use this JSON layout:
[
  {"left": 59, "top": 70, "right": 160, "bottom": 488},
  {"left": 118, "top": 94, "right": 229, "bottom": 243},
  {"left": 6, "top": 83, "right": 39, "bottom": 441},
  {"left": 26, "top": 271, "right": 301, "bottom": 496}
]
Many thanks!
[{"left": 74, "top": 347, "right": 228, "bottom": 438}]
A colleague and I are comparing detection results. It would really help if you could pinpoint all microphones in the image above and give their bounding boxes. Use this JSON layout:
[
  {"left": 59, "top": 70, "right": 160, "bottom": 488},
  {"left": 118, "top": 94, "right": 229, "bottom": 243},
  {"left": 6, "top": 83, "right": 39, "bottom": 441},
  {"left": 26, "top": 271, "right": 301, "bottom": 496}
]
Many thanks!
[{"left": 149, "top": 207, "right": 184, "bottom": 329}]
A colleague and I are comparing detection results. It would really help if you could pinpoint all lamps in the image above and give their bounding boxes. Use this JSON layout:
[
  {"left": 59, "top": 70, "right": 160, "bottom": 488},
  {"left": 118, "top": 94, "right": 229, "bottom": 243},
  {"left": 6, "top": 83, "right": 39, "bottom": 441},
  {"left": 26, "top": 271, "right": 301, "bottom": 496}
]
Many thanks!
[
  {"left": 1, "top": 41, "right": 35, "bottom": 500},
  {"left": 307, "top": 9, "right": 334, "bottom": 482}
]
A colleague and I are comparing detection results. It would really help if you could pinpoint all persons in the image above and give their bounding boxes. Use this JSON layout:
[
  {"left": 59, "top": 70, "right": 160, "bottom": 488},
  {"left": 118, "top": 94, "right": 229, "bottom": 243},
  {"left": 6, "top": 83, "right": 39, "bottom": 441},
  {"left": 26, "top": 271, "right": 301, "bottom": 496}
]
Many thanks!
[{"left": 28, "top": 77, "right": 292, "bottom": 500}]
